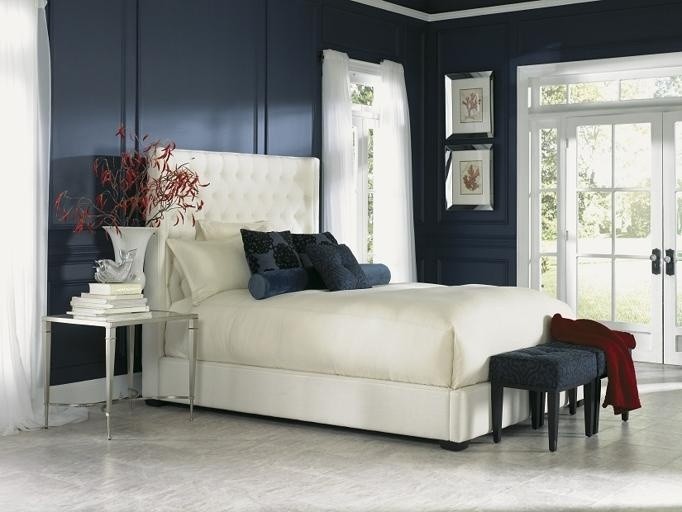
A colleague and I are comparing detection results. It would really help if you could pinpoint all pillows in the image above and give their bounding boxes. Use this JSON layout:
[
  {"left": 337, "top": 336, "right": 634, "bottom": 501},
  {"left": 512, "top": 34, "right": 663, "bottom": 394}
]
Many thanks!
[{"left": 167, "top": 217, "right": 391, "bottom": 306}]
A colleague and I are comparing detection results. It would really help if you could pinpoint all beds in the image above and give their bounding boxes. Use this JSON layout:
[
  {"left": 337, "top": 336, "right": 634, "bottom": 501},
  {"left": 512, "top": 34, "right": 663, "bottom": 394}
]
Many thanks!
[{"left": 141, "top": 144, "right": 585, "bottom": 452}]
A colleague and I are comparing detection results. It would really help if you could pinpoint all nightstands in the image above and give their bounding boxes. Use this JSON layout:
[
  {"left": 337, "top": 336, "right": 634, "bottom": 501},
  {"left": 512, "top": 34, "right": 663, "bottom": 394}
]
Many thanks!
[{"left": 40, "top": 310, "right": 199, "bottom": 440}]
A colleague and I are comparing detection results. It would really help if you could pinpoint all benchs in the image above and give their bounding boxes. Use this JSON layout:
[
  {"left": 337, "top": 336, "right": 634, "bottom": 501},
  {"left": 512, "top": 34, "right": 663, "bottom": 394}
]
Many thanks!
[{"left": 490, "top": 340, "right": 629, "bottom": 452}]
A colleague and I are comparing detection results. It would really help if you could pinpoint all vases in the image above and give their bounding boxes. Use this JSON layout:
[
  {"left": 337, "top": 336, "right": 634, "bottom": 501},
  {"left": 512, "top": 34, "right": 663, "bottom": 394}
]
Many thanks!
[{"left": 102, "top": 226, "right": 158, "bottom": 283}]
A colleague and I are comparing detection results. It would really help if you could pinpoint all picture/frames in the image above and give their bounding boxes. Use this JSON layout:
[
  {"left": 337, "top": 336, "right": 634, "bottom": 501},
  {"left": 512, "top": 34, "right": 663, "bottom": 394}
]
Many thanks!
[{"left": 443, "top": 70, "right": 496, "bottom": 214}]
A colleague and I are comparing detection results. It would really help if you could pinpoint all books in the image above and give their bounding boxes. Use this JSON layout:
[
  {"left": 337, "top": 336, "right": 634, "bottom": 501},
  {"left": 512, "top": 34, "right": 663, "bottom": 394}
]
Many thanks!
[{"left": 65, "top": 282, "right": 153, "bottom": 322}]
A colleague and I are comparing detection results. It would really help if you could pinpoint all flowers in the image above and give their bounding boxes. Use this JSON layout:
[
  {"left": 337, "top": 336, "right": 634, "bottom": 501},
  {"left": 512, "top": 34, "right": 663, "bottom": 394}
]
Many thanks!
[{"left": 51, "top": 121, "right": 212, "bottom": 240}]
